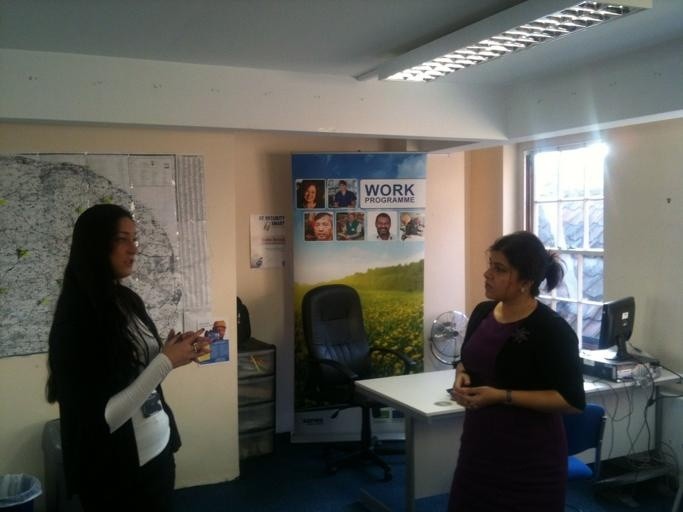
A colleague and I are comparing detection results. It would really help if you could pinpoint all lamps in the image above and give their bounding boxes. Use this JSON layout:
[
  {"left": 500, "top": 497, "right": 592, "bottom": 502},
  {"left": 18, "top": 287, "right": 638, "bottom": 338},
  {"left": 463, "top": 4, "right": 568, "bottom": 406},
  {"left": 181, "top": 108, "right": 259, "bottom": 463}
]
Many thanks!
[{"left": 378, "top": 0, "right": 652, "bottom": 82}]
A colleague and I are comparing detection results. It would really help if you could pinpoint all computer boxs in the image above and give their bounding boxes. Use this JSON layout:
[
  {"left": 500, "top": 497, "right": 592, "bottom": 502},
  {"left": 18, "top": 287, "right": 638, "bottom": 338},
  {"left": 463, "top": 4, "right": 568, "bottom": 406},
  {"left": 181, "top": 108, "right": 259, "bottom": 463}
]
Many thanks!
[{"left": 579, "top": 352, "right": 660, "bottom": 382}]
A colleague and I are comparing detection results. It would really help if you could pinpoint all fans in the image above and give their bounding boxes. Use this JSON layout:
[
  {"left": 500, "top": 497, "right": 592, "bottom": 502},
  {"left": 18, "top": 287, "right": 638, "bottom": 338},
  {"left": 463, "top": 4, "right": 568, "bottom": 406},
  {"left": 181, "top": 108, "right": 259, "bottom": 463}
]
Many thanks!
[{"left": 427, "top": 311, "right": 469, "bottom": 370}]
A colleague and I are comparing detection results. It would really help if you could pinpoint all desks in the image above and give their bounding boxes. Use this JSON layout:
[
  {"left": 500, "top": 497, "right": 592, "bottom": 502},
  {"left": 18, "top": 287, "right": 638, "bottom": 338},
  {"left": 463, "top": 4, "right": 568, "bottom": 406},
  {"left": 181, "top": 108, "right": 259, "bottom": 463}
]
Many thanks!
[{"left": 355, "top": 368, "right": 683, "bottom": 512}]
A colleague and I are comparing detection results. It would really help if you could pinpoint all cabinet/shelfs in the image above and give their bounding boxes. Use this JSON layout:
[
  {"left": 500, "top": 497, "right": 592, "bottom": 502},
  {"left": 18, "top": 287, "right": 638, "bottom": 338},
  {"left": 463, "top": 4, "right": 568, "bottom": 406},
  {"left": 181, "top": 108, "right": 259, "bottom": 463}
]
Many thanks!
[{"left": 238, "top": 337, "right": 277, "bottom": 461}]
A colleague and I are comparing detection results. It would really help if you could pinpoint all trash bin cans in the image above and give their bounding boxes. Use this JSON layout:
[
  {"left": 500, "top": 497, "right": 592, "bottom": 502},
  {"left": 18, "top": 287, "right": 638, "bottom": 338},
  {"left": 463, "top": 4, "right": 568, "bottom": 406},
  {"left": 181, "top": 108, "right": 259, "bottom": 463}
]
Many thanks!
[{"left": 0, "top": 475, "right": 39, "bottom": 512}]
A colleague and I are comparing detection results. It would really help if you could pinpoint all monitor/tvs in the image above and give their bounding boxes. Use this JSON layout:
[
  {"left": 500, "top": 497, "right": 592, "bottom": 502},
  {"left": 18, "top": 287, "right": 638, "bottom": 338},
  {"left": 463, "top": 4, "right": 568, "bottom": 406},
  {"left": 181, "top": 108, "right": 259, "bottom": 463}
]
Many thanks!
[{"left": 599, "top": 296, "right": 635, "bottom": 361}]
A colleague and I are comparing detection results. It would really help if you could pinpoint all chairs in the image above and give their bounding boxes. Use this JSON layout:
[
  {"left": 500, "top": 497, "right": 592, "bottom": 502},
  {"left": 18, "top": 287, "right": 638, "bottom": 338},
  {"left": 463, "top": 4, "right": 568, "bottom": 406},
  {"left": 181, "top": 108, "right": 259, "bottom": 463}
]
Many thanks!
[
  {"left": 561, "top": 403, "right": 607, "bottom": 512},
  {"left": 302, "top": 284, "right": 417, "bottom": 478}
]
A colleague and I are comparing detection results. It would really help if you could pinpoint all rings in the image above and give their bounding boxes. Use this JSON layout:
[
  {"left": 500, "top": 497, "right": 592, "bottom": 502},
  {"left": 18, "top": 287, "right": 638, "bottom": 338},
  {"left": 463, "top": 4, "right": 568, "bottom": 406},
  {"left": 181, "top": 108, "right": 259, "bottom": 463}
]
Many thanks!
[{"left": 191, "top": 344, "right": 198, "bottom": 352}]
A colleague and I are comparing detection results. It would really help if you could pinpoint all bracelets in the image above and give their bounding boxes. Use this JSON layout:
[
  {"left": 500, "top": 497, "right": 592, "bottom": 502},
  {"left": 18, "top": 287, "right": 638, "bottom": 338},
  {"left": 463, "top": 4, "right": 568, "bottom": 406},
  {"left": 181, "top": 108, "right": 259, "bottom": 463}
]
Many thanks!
[{"left": 504, "top": 387, "right": 512, "bottom": 406}]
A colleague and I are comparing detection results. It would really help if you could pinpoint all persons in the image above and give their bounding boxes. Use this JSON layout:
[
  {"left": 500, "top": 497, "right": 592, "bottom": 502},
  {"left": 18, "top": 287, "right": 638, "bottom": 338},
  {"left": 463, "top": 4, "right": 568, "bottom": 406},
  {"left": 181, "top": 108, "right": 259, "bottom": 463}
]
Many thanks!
[
  {"left": 300, "top": 182, "right": 320, "bottom": 208},
  {"left": 446, "top": 229, "right": 586, "bottom": 510},
  {"left": 42, "top": 203, "right": 227, "bottom": 511},
  {"left": 400, "top": 212, "right": 418, "bottom": 240},
  {"left": 312, "top": 212, "right": 333, "bottom": 240},
  {"left": 332, "top": 180, "right": 356, "bottom": 208},
  {"left": 336, "top": 213, "right": 364, "bottom": 240},
  {"left": 374, "top": 212, "right": 392, "bottom": 241}
]
